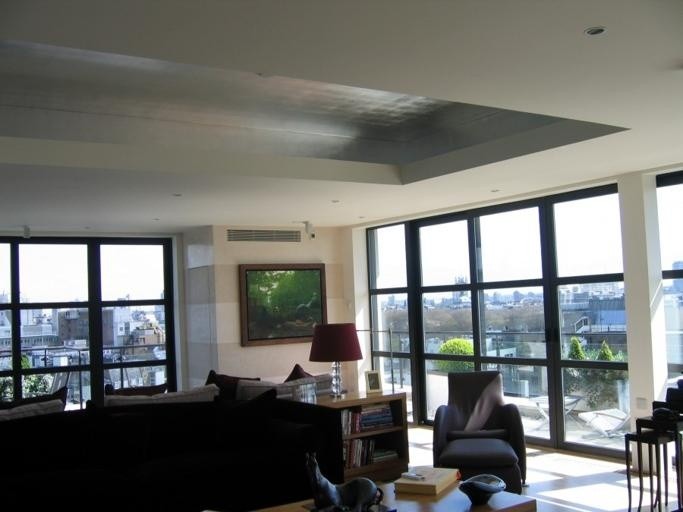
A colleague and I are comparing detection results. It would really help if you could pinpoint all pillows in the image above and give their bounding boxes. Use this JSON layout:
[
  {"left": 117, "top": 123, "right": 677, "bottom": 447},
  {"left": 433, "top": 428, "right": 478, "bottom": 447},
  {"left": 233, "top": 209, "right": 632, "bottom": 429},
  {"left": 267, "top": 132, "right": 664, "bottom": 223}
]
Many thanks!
[
  {"left": 105, "top": 383, "right": 168, "bottom": 406},
  {"left": 0, "top": 386, "right": 68, "bottom": 422},
  {"left": 205, "top": 370, "right": 260, "bottom": 399},
  {"left": 284, "top": 364, "right": 312, "bottom": 383}
]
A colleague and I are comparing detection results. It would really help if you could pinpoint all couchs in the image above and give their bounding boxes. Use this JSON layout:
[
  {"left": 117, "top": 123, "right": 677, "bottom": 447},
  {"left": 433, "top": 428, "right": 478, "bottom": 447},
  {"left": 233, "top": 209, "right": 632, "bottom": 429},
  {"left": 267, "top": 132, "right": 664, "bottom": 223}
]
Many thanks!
[{"left": 1, "top": 364, "right": 334, "bottom": 511}]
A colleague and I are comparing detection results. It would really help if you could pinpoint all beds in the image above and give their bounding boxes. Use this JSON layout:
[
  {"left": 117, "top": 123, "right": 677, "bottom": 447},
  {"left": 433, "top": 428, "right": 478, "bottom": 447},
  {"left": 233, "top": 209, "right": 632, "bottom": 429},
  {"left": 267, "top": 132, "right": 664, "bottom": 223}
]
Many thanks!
[{"left": 578, "top": 408, "right": 631, "bottom": 438}]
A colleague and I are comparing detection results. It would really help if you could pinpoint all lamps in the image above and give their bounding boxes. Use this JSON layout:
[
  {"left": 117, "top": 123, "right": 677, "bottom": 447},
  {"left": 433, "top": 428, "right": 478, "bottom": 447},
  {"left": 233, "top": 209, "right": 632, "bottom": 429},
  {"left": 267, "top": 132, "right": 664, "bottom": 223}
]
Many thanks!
[{"left": 308, "top": 323, "right": 363, "bottom": 397}]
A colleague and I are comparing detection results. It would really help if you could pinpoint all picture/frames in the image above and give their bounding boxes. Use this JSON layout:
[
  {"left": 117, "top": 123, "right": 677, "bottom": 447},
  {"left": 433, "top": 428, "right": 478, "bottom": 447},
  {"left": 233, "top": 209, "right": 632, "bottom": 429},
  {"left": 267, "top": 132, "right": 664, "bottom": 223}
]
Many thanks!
[
  {"left": 365, "top": 370, "right": 383, "bottom": 394},
  {"left": 238, "top": 263, "right": 328, "bottom": 347}
]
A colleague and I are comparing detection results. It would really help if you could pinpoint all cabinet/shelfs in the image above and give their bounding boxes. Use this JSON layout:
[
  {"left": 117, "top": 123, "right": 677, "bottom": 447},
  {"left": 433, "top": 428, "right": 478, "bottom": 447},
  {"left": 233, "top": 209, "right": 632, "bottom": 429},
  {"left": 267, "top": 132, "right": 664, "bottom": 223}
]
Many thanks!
[{"left": 277, "top": 391, "right": 409, "bottom": 486}]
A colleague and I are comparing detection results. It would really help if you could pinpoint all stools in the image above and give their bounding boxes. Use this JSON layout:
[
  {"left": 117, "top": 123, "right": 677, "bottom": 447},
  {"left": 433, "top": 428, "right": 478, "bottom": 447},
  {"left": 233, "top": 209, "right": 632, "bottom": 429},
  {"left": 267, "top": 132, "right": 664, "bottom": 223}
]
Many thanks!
[{"left": 624, "top": 430, "right": 683, "bottom": 512}]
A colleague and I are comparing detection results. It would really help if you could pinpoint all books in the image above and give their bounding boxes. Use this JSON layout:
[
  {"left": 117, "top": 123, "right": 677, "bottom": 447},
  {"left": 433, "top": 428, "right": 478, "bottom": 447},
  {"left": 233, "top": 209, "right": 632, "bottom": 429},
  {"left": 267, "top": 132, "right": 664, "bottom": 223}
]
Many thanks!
[
  {"left": 341, "top": 401, "right": 410, "bottom": 436},
  {"left": 342, "top": 437, "right": 400, "bottom": 472}
]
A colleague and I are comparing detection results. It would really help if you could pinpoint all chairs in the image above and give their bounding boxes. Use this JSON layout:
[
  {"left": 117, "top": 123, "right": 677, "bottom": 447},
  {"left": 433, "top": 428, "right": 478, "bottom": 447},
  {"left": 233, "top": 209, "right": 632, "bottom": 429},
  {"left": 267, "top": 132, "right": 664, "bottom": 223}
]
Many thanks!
[{"left": 432, "top": 371, "right": 526, "bottom": 495}]
